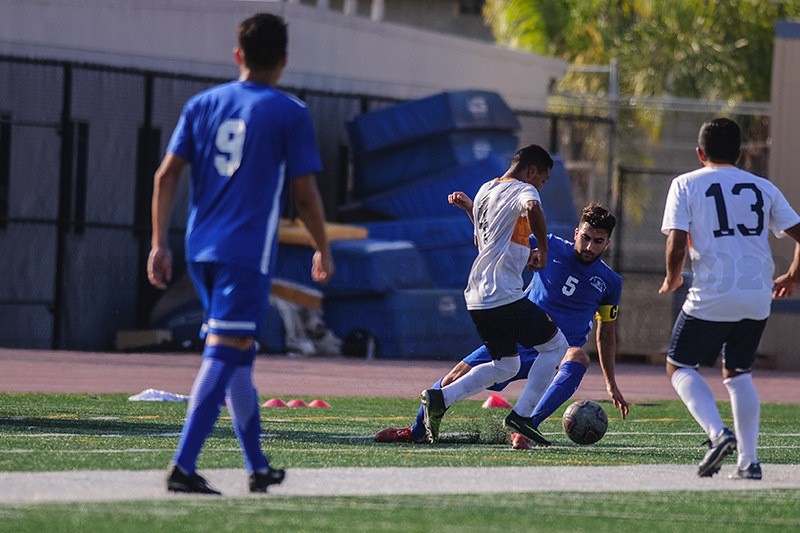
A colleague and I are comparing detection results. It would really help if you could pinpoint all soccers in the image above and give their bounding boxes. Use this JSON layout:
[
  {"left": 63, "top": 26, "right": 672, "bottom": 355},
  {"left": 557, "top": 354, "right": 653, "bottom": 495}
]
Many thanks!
[{"left": 562, "top": 399, "right": 609, "bottom": 445}]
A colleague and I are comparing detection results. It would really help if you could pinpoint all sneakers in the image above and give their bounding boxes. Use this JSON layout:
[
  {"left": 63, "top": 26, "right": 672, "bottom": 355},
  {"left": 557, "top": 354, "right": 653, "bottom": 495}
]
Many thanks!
[
  {"left": 697, "top": 428, "right": 737, "bottom": 478},
  {"left": 168, "top": 464, "right": 221, "bottom": 495},
  {"left": 249, "top": 467, "right": 285, "bottom": 491},
  {"left": 376, "top": 427, "right": 412, "bottom": 442},
  {"left": 419, "top": 388, "right": 451, "bottom": 443},
  {"left": 737, "top": 462, "right": 763, "bottom": 480},
  {"left": 510, "top": 432, "right": 530, "bottom": 449},
  {"left": 502, "top": 410, "right": 551, "bottom": 447}
]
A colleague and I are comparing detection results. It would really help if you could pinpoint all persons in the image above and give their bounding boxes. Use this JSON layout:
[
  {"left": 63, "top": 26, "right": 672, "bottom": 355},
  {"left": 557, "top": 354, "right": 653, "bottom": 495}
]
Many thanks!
[
  {"left": 148, "top": 12, "right": 335, "bottom": 496},
  {"left": 375, "top": 192, "right": 630, "bottom": 453},
  {"left": 419, "top": 143, "right": 570, "bottom": 447},
  {"left": 657, "top": 117, "right": 800, "bottom": 480}
]
